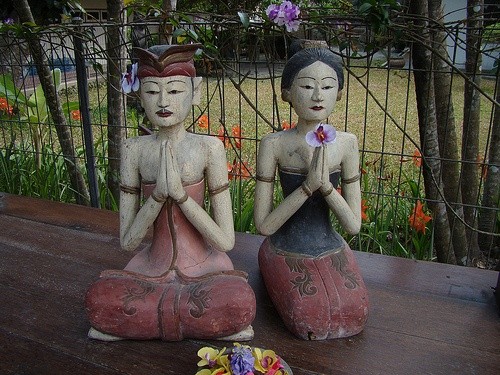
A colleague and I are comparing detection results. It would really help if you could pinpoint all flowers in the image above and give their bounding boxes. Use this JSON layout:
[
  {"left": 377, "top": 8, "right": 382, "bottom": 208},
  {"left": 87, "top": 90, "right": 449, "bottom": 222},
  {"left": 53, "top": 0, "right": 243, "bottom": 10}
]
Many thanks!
[
  {"left": 195, "top": 343, "right": 289, "bottom": 375},
  {"left": 120, "top": 63, "right": 139, "bottom": 94},
  {"left": 305, "top": 121, "right": 337, "bottom": 149}
]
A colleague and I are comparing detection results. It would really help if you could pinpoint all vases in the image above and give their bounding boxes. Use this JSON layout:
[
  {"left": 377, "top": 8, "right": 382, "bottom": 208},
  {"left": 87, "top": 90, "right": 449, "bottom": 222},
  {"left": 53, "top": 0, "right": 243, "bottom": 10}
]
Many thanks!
[{"left": 223, "top": 347, "right": 293, "bottom": 375}]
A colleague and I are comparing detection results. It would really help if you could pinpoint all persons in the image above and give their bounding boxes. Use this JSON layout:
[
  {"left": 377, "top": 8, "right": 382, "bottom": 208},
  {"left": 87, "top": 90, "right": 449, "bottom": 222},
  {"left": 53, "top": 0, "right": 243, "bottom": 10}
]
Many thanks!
[
  {"left": 254, "top": 39, "right": 369, "bottom": 342},
  {"left": 84, "top": 43, "right": 257, "bottom": 342}
]
